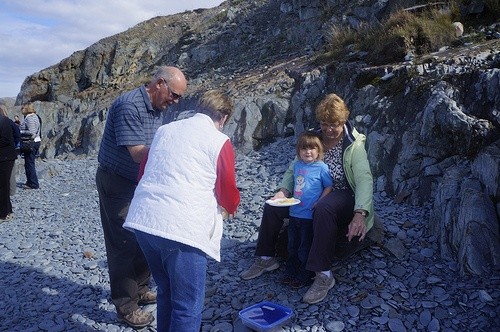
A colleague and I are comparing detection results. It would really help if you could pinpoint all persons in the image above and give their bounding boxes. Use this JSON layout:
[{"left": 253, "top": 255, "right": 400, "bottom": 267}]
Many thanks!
[
  {"left": 280, "top": 133, "right": 334, "bottom": 288},
  {"left": 240, "top": 93, "right": 374, "bottom": 303},
  {"left": 122, "top": 90, "right": 241, "bottom": 332},
  {"left": 96, "top": 67, "right": 187, "bottom": 328},
  {"left": 0, "top": 102, "right": 42, "bottom": 224}
]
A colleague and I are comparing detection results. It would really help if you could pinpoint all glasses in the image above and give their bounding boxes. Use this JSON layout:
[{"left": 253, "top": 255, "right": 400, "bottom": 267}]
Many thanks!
[
  {"left": 320, "top": 122, "right": 340, "bottom": 129},
  {"left": 164, "top": 79, "right": 181, "bottom": 100}
]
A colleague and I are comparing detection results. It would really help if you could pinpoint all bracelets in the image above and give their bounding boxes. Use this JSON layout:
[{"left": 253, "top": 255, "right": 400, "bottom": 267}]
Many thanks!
[{"left": 354, "top": 210, "right": 369, "bottom": 217}]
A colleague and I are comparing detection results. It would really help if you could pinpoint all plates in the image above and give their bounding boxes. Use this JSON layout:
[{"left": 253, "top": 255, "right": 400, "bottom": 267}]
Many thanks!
[{"left": 265, "top": 198, "right": 301, "bottom": 207}]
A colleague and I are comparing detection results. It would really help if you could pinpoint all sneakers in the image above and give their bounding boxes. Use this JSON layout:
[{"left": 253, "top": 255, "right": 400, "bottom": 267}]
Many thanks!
[
  {"left": 302, "top": 270, "right": 336, "bottom": 303},
  {"left": 239, "top": 256, "right": 279, "bottom": 280}
]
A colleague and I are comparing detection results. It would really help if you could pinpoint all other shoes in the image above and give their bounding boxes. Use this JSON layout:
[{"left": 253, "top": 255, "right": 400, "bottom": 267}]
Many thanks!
[
  {"left": 118, "top": 307, "right": 155, "bottom": 328},
  {"left": 0, "top": 212, "right": 13, "bottom": 222},
  {"left": 139, "top": 288, "right": 158, "bottom": 304},
  {"left": 22, "top": 184, "right": 33, "bottom": 188}
]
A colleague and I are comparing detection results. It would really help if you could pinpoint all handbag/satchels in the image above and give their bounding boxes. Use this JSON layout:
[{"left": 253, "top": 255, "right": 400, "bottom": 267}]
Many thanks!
[{"left": 20, "top": 134, "right": 34, "bottom": 151}]
